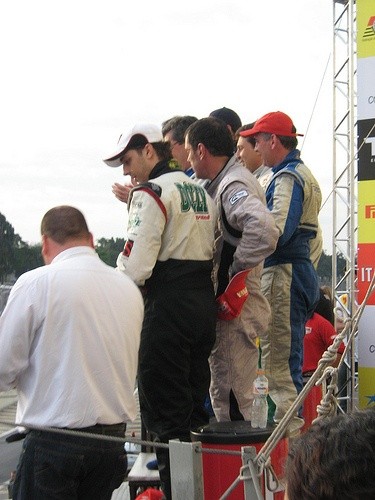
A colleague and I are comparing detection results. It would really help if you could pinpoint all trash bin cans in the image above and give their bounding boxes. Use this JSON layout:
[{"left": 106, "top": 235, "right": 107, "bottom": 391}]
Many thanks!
[{"left": 191, "top": 421, "right": 289, "bottom": 500}]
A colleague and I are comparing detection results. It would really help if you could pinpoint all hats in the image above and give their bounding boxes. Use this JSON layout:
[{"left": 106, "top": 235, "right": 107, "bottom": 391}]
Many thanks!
[
  {"left": 215, "top": 270, "right": 249, "bottom": 321},
  {"left": 102, "top": 122, "right": 163, "bottom": 167},
  {"left": 209, "top": 107, "right": 242, "bottom": 132},
  {"left": 239, "top": 111, "right": 304, "bottom": 136}
]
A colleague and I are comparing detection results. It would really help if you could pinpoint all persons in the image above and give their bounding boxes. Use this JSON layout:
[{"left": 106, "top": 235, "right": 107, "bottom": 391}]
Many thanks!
[
  {"left": 284, "top": 405, "right": 375, "bottom": 499},
  {"left": 102, "top": 107, "right": 358, "bottom": 471},
  {"left": 0, "top": 206, "right": 145, "bottom": 500}
]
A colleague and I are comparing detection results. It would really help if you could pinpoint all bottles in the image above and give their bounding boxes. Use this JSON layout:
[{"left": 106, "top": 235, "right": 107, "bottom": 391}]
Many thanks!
[{"left": 251, "top": 370, "right": 268, "bottom": 429}]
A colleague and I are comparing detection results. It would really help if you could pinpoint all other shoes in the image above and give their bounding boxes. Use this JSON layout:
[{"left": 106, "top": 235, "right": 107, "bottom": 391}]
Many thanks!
[{"left": 147, "top": 461, "right": 159, "bottom": 470}]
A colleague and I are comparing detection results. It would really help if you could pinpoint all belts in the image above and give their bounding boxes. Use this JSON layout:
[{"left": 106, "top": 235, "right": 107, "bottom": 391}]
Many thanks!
[{"left": 6, "top": 423, "right": 126, "bottom": 444}]
